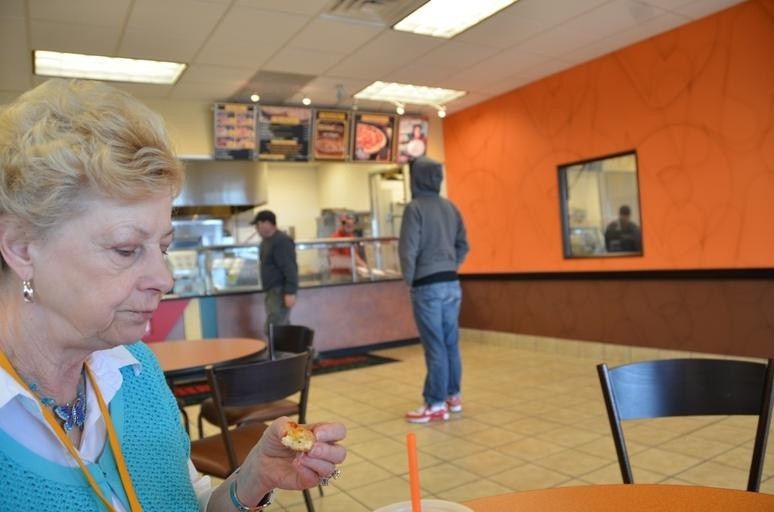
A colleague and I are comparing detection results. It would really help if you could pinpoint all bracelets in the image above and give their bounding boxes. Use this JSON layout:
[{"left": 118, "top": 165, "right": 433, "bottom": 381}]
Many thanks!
[{"left": 228, "top": 464, "right": 277, "bottom": 511}]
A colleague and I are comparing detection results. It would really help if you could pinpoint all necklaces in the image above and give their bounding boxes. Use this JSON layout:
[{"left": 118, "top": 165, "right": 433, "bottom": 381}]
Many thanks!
[{"left": 12, "top": 365, "right": 89, "bottom": 434}]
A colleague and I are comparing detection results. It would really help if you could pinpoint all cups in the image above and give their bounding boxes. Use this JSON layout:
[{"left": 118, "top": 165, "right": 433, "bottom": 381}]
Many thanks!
[{"left": 371, "top": 499, "right": 475, "bottom": 512}]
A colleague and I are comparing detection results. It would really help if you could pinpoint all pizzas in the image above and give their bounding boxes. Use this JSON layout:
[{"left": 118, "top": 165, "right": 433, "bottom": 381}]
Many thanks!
[
  {"left": 357, "top": 122, "right": 387, "bottom": 155},
  {"left": 279, "top": 420, "right": 313, "bottom": 454}
]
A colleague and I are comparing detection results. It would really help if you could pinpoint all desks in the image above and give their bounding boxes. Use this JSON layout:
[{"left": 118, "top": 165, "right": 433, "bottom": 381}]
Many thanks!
[{"left": 144, "top": 337, "right": 268, "bottom": 435}]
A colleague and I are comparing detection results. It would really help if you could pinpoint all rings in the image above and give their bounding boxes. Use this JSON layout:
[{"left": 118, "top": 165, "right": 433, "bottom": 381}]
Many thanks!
[{"left": 328, "top": 467, "right": 342, "bottom": 481}]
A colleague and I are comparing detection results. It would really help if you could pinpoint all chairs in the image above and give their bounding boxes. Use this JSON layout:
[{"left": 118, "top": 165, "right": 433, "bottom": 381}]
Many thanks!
[
  {"left": 596, "top": 357, "right": 773, "bottom": 492},
  {"left": 189, "top": 323, "right": 322, "bottom": 511}
]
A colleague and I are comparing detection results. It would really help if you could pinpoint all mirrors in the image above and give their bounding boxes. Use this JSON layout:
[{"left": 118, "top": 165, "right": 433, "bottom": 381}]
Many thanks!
[{"left": 556, "top": 149, "right": 642, "bottom": 259}]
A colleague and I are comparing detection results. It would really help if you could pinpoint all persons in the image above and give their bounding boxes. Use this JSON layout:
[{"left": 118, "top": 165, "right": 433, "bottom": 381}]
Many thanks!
[
  {"left": 395, "top": 156, "right": 470, "bottom": 427},
  {"left": 602, "top": 205, "right": 641, "bottom": 253},
  {"left": 403, "top": 122, "right": 427, "bottom": 156},
  {"left": 248, "top": 209, "right": 298, "bottom": 335},
  {"left": 0, "top": 75, "right": 349, "bottom": 511},
  {"left": 327, "top": 212, "right": 370, "bottom": 279}
]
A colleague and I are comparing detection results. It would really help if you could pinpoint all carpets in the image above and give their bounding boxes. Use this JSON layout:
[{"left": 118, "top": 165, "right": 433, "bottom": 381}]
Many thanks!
[{"left": 310, "top": 352, "right": 401, "bottom": 375}]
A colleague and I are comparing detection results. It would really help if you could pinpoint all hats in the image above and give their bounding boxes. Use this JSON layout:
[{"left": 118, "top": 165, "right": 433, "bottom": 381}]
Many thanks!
[{"left": 250, "top": 211, "right": 275, "bottom": 225}]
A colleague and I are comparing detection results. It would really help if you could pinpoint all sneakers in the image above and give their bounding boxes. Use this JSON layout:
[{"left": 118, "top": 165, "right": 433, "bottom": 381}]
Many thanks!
[{"left": 404, "top": 392, "right": 462, "bottom": 423}]
